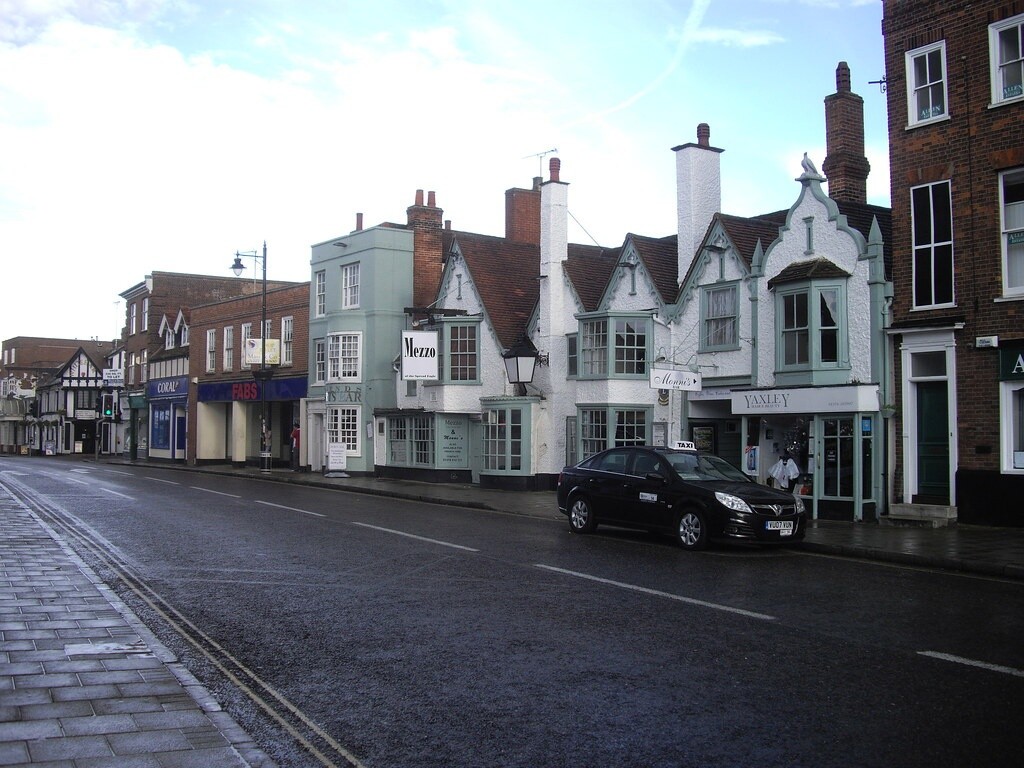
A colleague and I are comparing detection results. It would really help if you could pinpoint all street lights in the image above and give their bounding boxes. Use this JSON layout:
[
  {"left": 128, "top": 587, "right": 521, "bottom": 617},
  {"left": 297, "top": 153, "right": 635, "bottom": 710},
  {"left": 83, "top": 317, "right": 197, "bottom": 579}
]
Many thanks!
[{"left": 229, "top": 240, "right": 267, "bottom": 452}]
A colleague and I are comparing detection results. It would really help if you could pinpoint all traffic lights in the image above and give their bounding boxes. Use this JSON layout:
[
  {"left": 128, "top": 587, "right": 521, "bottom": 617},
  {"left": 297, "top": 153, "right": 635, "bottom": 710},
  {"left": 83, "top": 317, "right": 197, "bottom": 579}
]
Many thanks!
[
  {"left": 95, "top": 396, "right": 103, "bottom": 414},
  {"left": 114, "top": 400, "right": 120, "bottom": 415},
  {"left": 103, "top": 394, "right": 114, "bottom": 418},
  {"left": 29, "top": 398, "right": 41, "bottom": 418}
]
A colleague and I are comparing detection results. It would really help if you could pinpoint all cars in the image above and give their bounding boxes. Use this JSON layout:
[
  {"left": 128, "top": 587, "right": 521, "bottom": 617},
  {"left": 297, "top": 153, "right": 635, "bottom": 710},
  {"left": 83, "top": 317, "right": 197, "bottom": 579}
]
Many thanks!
[{"left": 557, "top": 440, "right": 807, "bottom": 553}]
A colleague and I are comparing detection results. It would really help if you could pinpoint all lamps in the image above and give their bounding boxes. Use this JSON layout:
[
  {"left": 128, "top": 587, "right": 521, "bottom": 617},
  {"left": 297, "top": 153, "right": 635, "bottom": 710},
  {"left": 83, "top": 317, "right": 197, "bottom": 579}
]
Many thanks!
[
  {"left": 502, "top": 337, "right": 549, "bottom": 384},
  {"left": 333, "top": 242, "right": 347, "bottom": 247},
  {"left": 656, "top": 346, "right": 700, "bottom": 374}
]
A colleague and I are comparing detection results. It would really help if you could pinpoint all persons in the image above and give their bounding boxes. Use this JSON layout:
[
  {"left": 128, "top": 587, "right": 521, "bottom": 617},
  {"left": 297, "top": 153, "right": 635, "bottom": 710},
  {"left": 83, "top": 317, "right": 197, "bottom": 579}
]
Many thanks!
[
  {"left": 290, "top": 423, "right": 300, "bottom": 470},
  {"left": 265, "top": 425, "right": 271, "bottom": 451}
]
